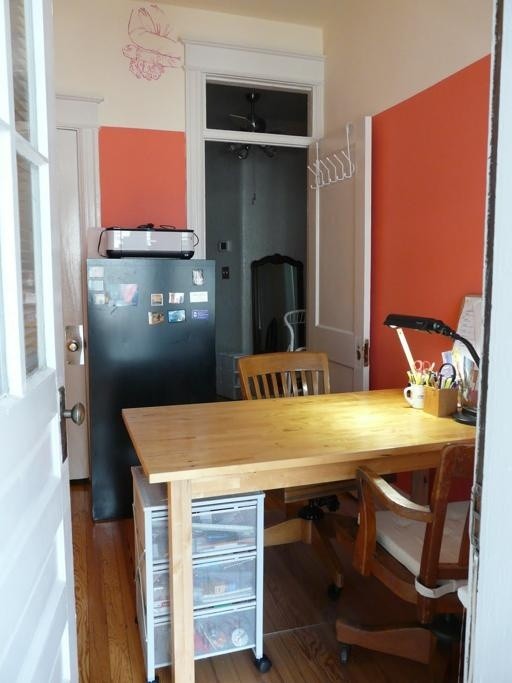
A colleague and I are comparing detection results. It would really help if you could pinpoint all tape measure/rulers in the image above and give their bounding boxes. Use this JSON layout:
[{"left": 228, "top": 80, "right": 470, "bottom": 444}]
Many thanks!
[{"left": 396, "top": 327, "right": 416, "bottom": 374}]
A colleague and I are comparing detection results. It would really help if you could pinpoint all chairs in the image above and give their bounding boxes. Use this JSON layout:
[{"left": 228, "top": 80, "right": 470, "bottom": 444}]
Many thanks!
[
  {"left": 234, "top": 352, "right": 362, "bottom": 595},
  {"left": 335, "top": 442, "right": 471, "bottom": 669}
]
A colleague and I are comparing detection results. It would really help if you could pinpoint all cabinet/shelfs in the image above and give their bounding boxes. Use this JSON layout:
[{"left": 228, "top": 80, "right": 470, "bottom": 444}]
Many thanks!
[{"left": 126, "top": 465, "right": 272, "bottom": 683}]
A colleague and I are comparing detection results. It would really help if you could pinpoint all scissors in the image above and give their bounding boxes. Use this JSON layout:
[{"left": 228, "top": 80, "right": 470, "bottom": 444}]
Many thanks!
[{"left": 414, "top": 360, "right": 430, "bottom": 375}]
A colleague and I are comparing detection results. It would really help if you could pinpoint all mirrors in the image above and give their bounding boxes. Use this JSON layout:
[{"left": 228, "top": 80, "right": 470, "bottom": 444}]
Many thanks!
[{"left": 248, "top": 251, "right": 305, "bottom": 354}]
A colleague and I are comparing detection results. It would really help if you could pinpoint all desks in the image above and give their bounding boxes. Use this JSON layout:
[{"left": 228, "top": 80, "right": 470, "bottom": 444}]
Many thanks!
[{"left": 121, "top": 386, "right": 477, "bottom": 683}]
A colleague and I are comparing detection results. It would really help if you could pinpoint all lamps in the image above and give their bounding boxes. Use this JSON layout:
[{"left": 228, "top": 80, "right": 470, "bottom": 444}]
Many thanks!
[{"left": 384, "top": 312, "right": 481, "bottom": 425}]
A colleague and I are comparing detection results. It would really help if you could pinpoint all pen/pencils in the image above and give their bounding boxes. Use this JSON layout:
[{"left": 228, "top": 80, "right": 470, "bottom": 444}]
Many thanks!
[{"left": 407, "top": 360, "right": 459, "bottom": 389}]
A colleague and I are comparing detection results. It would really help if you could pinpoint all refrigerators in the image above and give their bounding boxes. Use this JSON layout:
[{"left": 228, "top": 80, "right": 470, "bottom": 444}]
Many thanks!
[{"left": 84, "top": 257, "right": 219, "bottom": 521}]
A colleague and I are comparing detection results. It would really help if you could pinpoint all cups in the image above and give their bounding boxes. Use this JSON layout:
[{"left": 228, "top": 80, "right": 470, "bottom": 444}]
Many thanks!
[{"left": 404, "top": 383, "right": 426, "bottom": 409}]
